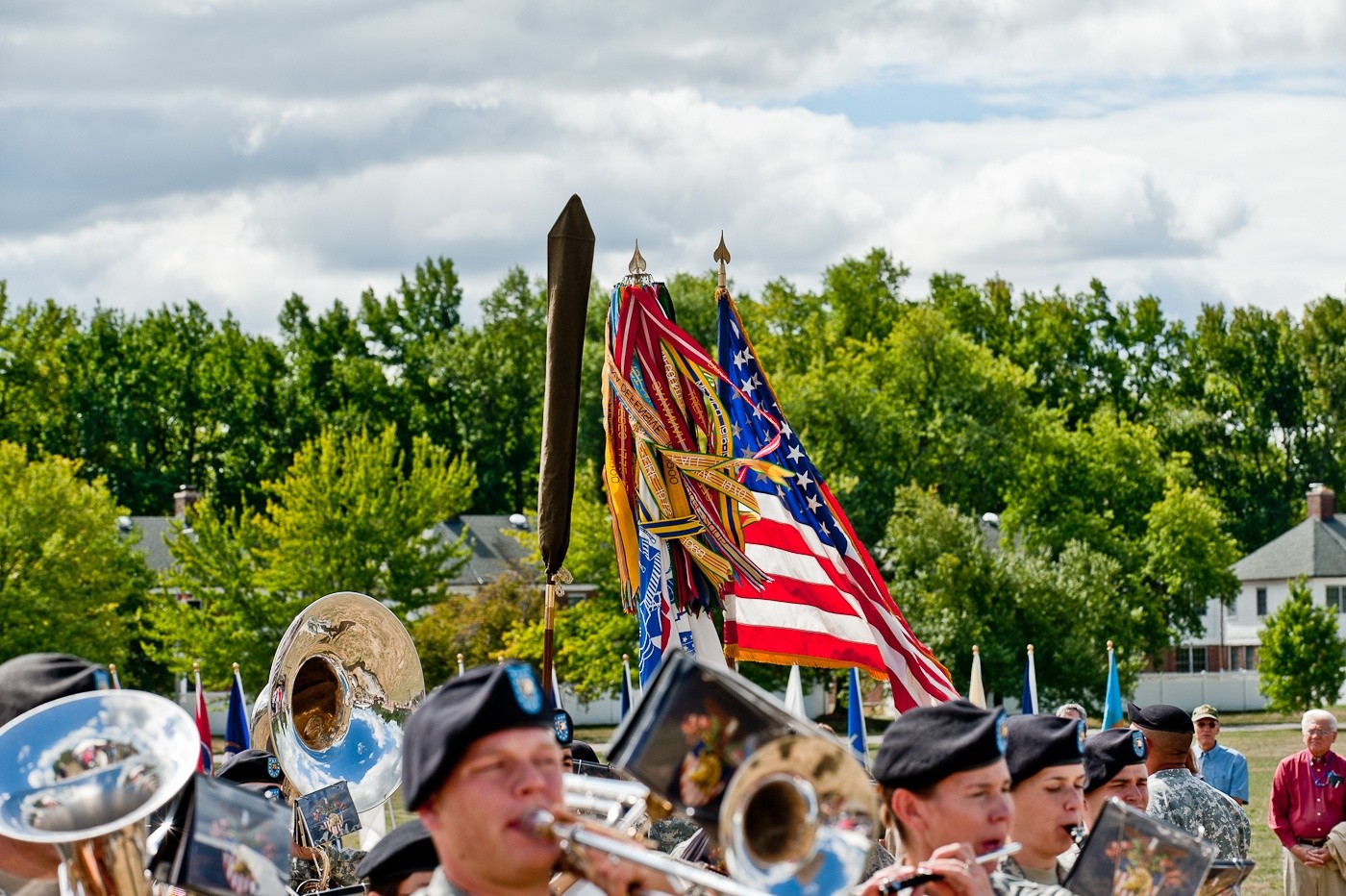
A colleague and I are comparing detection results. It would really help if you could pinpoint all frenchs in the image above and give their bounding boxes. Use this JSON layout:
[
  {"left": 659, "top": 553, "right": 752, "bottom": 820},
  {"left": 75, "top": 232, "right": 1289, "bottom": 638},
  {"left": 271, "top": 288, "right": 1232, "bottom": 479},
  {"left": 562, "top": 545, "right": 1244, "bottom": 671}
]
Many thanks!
[{"left": 251, "top": 592, "right": 427, "bottom": 896}]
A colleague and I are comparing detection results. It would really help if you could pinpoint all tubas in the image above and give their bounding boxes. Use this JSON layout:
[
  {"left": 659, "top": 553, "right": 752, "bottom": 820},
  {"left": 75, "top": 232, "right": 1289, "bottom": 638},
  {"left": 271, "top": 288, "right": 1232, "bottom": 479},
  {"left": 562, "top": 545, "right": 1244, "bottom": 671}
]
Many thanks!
[
  {"left": 0, "top": 689, "right": 198, "bottom": 896},
  {"left": 562, "top": 772, "right": 678, "bottom": 837}
]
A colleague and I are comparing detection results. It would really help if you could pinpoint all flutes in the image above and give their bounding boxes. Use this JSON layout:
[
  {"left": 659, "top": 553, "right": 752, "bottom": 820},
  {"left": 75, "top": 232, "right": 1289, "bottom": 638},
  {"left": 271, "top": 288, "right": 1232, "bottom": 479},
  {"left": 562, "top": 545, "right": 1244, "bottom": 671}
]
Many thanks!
[
  {"left": 879, "top": 842, "right": 1024, "bottom": 895},
  {"left": 1066, "top": 823, "right": 1091, "bottom": 853}
]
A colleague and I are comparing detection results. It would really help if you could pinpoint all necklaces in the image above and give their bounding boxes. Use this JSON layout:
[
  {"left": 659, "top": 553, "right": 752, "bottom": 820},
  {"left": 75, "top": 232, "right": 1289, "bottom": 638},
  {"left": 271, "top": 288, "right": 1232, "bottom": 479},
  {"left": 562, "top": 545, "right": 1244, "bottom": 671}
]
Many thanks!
[{"left": 1306, "top": 751, "right": 1332, "bottom": 786}]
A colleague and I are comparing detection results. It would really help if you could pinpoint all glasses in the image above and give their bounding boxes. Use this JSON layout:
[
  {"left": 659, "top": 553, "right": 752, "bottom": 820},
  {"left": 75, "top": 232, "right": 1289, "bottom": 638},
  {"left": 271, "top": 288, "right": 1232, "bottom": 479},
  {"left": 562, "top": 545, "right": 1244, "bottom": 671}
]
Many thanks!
[
  {"left": 1197, "top": 721, "right": 1216, "bottom": 728},
  {"left": 1307, "top": 729, "right": 1330, "bottom": 737}
]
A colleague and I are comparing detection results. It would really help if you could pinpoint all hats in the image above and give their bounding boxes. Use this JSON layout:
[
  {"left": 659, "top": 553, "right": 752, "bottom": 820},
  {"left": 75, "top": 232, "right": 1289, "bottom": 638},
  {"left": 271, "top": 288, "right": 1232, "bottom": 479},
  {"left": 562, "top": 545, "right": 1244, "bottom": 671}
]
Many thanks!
[
  {"left": 0, "top": 649, "right": 105, "bottom": 733},
  {"left": 870, "top": 701, "right": 1000, "bottom": 789},
  {"left": 399, "top": 655, "right": 555, "bottom": 814},
  {"left": 213, "top": 749, "right": 286, "bottom": 788},
  {"left": 999, "top": 714, "right": 1083, "bottom": 789},
  {"left": 1191, "top": 705, "right": 1219, "bottom": 722},
  {"left": 353, "top": 820, "right": 442, "bottom": 889},
  {"left": 565, "top": 737, "right": 600, "bottom": 775},
  {"left": 553, "top": 708, "right": 574, "bottom": 748},
  {"left": 1082, "top": 727, "right": 1149, "bottom": 791},
  {"left": 1127, "top": 700, "right": 1196, "bottom": 733}
]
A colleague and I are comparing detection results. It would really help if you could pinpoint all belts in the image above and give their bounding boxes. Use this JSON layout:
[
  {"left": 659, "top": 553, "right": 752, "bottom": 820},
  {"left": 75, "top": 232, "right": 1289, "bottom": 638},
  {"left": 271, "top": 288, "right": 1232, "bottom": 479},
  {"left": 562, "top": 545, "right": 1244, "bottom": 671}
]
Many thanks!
[{"left": 1296, "top": 837, "right": 1327, "bottom": 848}]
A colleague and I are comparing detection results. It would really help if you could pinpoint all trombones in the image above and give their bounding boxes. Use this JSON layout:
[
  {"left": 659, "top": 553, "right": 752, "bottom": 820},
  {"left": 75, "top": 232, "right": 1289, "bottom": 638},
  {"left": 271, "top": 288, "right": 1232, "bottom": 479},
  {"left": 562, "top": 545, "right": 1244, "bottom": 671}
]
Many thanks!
[{"left": 519, "top": 736, "right": 880, "bottom": 896}]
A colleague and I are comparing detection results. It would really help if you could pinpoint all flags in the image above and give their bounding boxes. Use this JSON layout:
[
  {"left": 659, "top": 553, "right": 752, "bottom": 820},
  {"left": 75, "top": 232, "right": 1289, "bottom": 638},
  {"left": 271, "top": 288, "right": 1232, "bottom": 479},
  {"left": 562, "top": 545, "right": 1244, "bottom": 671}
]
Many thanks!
[
  {"left": 622, "top": 662, "right": 633, "bottom": 718},
  {"left": 458, "top": 661, "right": 564, "bottom": 709},
  {"left": 112, "top": 675, "right": 120, "bottom": 688},
  {"left": 1022, "top": 652, "right": 1040, "bottom": 715},
  {"left": 970, "top": 653, "right": 986, "bottom": 709},
  {"left": 848, "top": 667, "right": 866, "bottom": 767},
  {"left": 717, "top": 295, "right": 960, "bottom": 719},
  {"left": 601, "top": 282, "right": 780, "bottom": 697},
  {"left": 1102, "top": 652, "right": 1124, "bottom": 730},
  {"left": 784, "top": 665, "right": 804, "bottom": 715},
  {"left": 195, "top": 674, "right": 214, "bottom": 776},
  {"left": 224, "top": 671, "right": 249, "bottom": 760}
]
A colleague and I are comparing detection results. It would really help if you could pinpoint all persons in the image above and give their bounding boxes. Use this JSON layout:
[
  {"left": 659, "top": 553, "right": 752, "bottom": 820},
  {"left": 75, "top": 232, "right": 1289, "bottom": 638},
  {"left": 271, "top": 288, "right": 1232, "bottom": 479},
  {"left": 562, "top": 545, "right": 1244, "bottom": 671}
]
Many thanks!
[
  {"left": 0, "top": 651, "right": 1257, "bottom": 896},
  {"left": 1267, "top": 709, "right": 1346, "bottom": 896}
]
